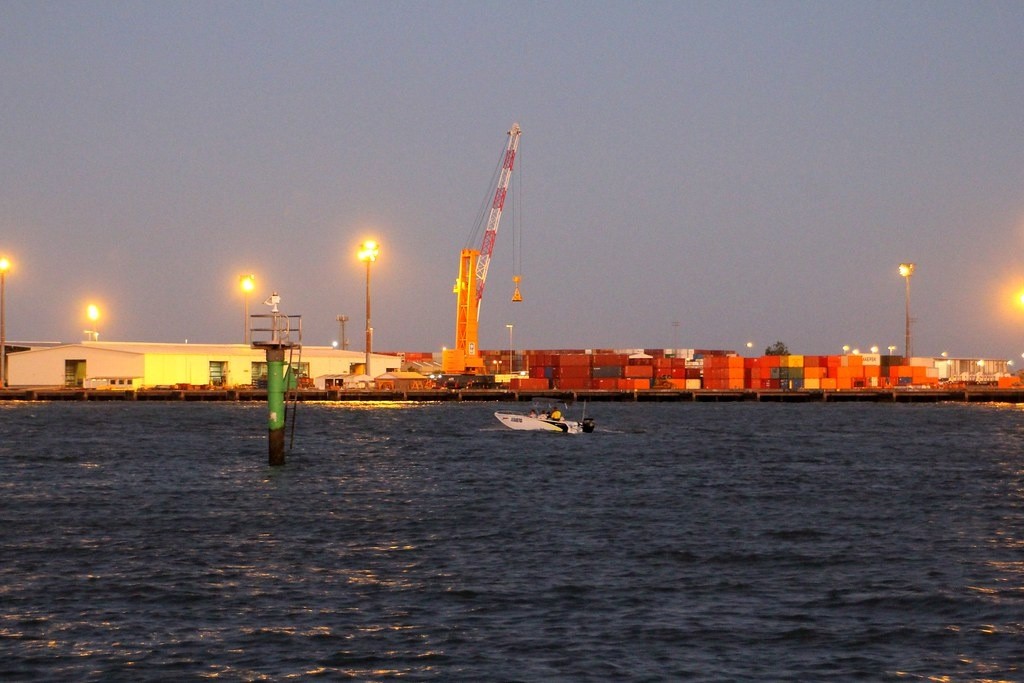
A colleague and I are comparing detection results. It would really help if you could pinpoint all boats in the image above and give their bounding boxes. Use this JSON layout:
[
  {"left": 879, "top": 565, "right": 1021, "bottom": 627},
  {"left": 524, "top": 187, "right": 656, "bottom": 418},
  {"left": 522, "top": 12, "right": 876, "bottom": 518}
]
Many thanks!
[{"left": 494, "top": 410, "right": 594, "bottom": 434}]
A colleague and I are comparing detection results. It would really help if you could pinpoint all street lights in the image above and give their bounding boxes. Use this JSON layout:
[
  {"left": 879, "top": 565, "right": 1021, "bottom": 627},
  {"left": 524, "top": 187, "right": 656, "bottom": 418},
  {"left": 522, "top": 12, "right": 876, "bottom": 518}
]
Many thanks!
[
  {"left": 336, "top": 315, "right": 349, "bottom": 350},
  {"left": 239, "top": 276, "right": 256, "bottom": 345},
  {"left": 355, "top": 239, "right": 378, "bottom": 376},
  {"left": 505, "top": 325, "right": 514, "bottom": 373},
  {"left": 899, "top": 262, "right": 916, "bottom": 359},
  {"left": 887, "top": 346, "right": 897, "bottom": 355},
  {"left": 0, "top": 258, "right": 9, "bottom": 389}
]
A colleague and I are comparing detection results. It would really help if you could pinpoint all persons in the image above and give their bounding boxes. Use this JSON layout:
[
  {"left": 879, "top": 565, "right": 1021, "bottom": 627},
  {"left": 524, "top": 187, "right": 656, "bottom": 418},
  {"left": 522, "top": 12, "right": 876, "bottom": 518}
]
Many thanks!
[
  {"left": 538, "top": 411, "right": 547, "bottom": 420},
  {"left": 551, "top": 407, "right": 561, "bottom": 421},
  {"left": 529, "top": 410, "right": 537, "bottom": 418}
]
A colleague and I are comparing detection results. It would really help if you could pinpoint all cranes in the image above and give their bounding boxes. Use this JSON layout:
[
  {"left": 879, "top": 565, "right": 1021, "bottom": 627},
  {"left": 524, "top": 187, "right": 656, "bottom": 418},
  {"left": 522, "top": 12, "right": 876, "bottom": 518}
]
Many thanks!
[{"left": 453, "top": 121, "right": 523, "bottom": 377}]
